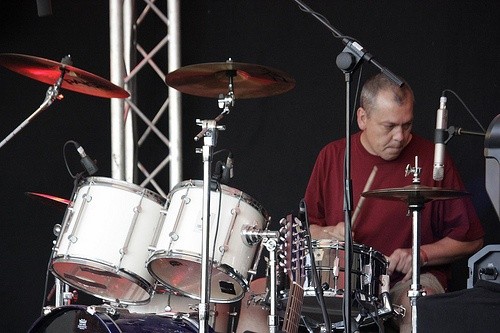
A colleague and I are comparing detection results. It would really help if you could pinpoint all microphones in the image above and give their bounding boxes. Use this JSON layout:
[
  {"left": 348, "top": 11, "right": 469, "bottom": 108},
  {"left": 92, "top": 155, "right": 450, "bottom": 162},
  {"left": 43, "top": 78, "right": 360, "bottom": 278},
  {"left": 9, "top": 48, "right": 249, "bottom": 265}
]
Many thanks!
[
  {"left": 74, "top": 142, "right": 102, "bottom": 176},
  {"left": 227, "top": 152, "right": 234, "bottom": 179},
  {"left": 432, "top": 96, "right": 448, "bottom": 182}
]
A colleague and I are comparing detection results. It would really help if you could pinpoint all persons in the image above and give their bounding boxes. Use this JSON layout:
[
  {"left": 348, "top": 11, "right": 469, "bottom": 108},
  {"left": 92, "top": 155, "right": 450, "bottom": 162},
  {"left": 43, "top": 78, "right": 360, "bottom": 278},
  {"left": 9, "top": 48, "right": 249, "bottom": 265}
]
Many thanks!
[{"left": 235, "top": 71, "right": 486, "bottom": 333}]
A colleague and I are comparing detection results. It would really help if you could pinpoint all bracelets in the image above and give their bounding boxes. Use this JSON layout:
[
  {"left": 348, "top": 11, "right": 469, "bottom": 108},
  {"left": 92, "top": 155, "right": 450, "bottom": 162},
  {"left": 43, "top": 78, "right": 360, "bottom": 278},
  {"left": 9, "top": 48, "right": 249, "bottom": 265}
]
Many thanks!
[{"left": 419, "top": 248, "right": 428, "bottom": 268}]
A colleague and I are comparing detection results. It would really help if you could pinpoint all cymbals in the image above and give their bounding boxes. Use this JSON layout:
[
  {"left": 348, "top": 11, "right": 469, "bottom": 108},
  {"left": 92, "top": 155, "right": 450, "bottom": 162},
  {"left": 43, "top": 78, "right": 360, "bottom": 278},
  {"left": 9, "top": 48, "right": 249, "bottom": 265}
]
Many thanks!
[
  {"left": 22, "top": 190, "right": 72, "bottom": 208},
  {"left": 0, "top": 52, "right": 131, "bottom": 99},
  {"left": 360, "top": 184, "right": 467, "bottom": 201},
  {"left": 165, "top": 60, "right": 297, "bottom": 100}
]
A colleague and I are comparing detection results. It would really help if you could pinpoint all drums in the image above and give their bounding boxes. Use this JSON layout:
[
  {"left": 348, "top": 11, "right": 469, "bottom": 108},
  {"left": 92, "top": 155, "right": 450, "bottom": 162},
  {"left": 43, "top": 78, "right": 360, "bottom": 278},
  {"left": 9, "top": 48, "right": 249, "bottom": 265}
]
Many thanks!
[
  {"left": 280, "top": 238, "right": 391, "bottom": 319},
  {"left": 49, "top": 176, "right": 169, "bottom": 306},
  {"left": 104, "top": 279, "right": 233, "bottom": 333},
  {"left": 26, "top": 304, "right": 223, "bottom": 332},
  {"left": 145, "top": 179, "right": 270, "bottom": 304}
]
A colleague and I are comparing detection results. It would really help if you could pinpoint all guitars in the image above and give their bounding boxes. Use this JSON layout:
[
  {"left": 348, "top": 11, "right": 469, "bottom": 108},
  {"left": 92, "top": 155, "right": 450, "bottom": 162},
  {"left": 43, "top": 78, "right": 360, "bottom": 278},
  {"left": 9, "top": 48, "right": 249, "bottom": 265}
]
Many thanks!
[{"left": 277, "top": 213, "right": 310, "bottom": 333}]
